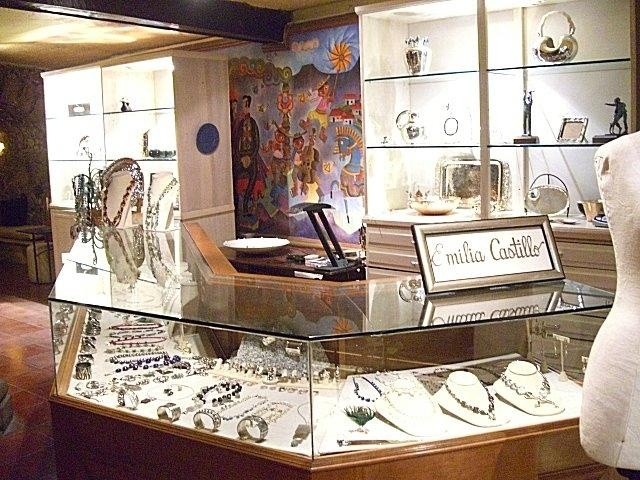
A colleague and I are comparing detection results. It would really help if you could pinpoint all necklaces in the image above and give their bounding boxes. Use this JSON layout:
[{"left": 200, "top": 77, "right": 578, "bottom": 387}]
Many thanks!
[
  {"left": 381, "top": 387, "right": 437, "bottom": 428},
  {"left": 104, "top": 320, "right": 296, "bottom": 427},
  {"left": 445, "top": 382, "right": 497, "bottom": 422},
  {"left": 99, "top": 170, "right": 137, "bottom": 226},
  {"left": 352, "top": 375, "right": 383, "bottom": 403},
  {"left": 143, "top": 177, "right": 178, "bottom": 229},
  {"left": 497, "top": 371, "right": 559, "bottom": 409},
  {"left": 96, "top": 231, "right": 176, "bottom": 282}
]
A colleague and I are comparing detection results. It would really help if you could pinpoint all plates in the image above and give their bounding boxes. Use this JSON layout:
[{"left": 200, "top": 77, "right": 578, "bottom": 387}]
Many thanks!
[{"left": 223, "top": 237, "right": 290, "bottom": 256}]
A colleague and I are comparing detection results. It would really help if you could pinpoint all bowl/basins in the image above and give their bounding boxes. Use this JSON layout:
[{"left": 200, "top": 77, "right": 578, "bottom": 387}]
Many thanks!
[{"left": 408, "top": 194, "right": 462, "bottom": 216}]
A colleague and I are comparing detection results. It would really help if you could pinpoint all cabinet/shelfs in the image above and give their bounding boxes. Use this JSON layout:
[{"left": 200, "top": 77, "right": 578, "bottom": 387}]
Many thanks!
[
  {"left": 352, "top": 1, "right": 639, "bottom": 375},
  {"left": 45, "top": 226, "right": 619, "bottom": 480},
  {"left": 38, "top": 49, "right": 239, "bottom": 282}
]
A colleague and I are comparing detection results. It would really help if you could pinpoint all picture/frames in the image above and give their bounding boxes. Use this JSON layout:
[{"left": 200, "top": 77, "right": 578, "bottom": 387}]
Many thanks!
[{"left": 556, "top": 116, "right": 589, "bottom": 144}]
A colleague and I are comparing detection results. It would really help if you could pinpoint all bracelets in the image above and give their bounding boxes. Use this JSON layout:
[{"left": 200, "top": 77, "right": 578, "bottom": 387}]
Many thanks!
[
  {"left": 236, "top": 415, "right": 269, "bottom": 443},
  {"left": 73, "top": 313, "right": 102, "bottom": 379},
  {"left": 53, "top": 301, "right": 75, "bottom": 355},
  {"left": 191, "top": 409, "right": 222, "bottom": 431}
]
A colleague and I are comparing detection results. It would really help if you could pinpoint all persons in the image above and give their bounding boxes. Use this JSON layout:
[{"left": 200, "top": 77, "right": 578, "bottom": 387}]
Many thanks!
[
  {"left": 522, "top": 89, "right": 533, "bottom": 136},
  {"left": 604, "top": 97, "right": 631, "bottom": 136},
  {"left": 578, "top": 130, "right": 640, "bottom": 473}
]
